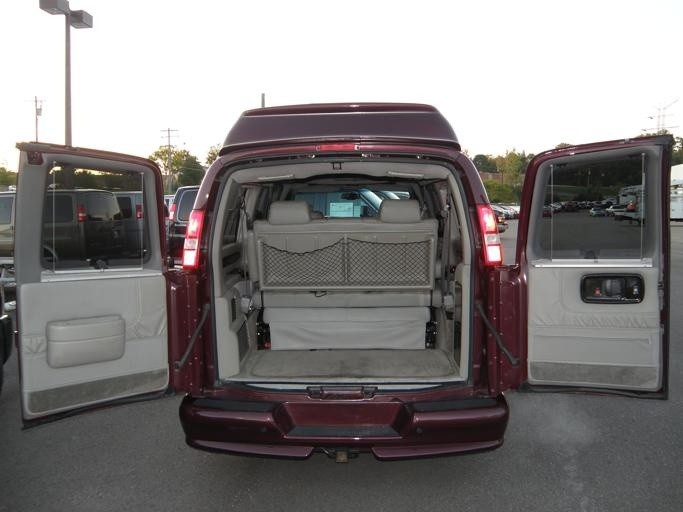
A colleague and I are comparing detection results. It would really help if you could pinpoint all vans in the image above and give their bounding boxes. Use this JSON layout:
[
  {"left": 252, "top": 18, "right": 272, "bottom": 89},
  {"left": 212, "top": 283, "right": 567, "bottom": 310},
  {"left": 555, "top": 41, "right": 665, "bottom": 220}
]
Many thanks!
[
  {"left": 1, "top": 188, "right": 128, "bottom": 294},
  {"left": 9, "top": 102, "right": 677, "bottom": 461},
  {"left": 111, "top": 191, "right": 145, "bottom": 260},
  {"left": 163, "top": 186, "right": 198, "bottom": 258}
]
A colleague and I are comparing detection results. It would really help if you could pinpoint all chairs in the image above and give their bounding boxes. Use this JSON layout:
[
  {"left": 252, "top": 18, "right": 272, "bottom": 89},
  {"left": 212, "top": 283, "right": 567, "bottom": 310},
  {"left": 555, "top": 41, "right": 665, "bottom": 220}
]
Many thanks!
[{"left": 247, "top": 200, "right": 438, "bottom": 308}]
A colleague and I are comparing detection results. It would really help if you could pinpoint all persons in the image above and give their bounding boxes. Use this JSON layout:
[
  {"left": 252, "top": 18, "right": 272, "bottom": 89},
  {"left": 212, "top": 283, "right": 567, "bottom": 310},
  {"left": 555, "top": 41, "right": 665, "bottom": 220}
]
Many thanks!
[
  {"left": 99, "top": 223, "right": 114, "bottom": 264},
  {"left": 627, "top": 201, "right": 635, "bottom": 223}
]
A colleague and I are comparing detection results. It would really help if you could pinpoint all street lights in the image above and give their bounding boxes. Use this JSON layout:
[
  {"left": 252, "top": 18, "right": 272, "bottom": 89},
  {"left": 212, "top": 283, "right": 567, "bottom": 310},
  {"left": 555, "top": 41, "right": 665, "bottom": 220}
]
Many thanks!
[{"left": 40, "top": 0, "right": 94, "bottom": 148}]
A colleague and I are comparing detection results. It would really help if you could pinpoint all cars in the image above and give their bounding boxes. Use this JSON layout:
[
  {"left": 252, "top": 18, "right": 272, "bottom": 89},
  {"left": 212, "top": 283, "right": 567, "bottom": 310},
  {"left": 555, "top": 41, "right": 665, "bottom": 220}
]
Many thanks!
[{"left": 485, "top": 185, "right": 646, "bottom": 246}]
